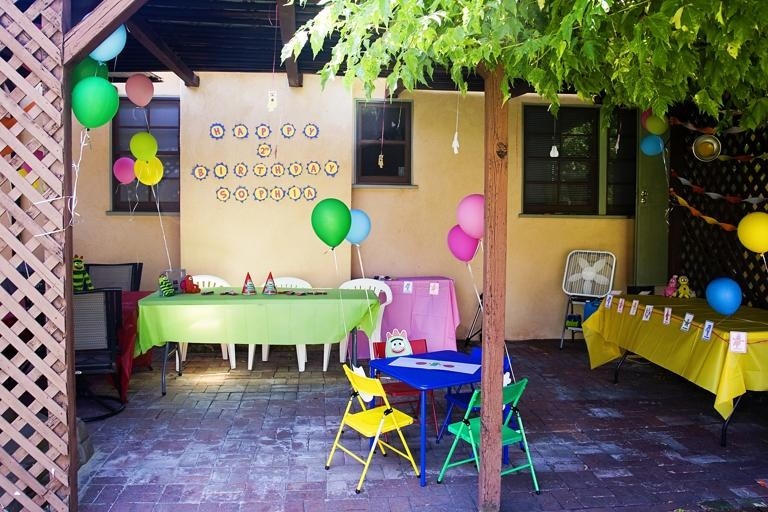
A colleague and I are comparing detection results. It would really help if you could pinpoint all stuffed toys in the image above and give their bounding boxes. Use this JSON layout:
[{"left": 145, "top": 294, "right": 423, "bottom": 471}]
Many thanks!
[
  {"left": 180, "top": 275, "right": 200, "bottom": 293},
  {"left": 159, "top": 274, "right": 175, "bottom": 297},
  {"left": 72, "top": 255, "right": 94, "bottom": 292}
]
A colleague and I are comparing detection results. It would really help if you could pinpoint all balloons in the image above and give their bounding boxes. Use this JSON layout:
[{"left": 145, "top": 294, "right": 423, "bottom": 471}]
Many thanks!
[
  {"left": 311, "top": 198, "right": 351, "bottom": 250},
  {"left": 455, "top": 193, "right": 484, "bottom": 239},
  {"left": 88, "top": 23, "right": 128, "bottom": 63},
  {"left": 447, "top": 224, "right": 480, "bottom": 262},
  {"left": 0, "top": 24, "right": 47, "bottom": 189},
  {"left": 736, "top": 211, "right": 768, "bottom": 257},
  {"left": 125, "top": 73, "right": 155, "bottom": 109},
  {"left": 345, "top": 209, "right": 371, "bottom": 245},
  {"left": 639, "top": 108, "right": 670, "bottom": 158},
  {"left": 70, "top": 76, "right": 120, "bottom": 130},
  {"left": 705, "top": 277, "right": 743, "bottom": 315},
  {"left": 112, "top": 132, "right": 164, "bottom": 187},
  {"left": 70, "top": 56, "right": 109, "bottom": 93}
]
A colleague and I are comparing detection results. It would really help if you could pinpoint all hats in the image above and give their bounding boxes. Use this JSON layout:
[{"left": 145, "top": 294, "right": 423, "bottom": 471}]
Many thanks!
[
  {"left": 262, "top": 272, "right": 277, "bottom": 294},
  {"left": 242, "top": 272, "right": 256, "bottom": 295}
]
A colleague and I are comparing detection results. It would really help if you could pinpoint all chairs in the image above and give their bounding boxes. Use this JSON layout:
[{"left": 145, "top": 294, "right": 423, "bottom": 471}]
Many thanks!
[
  {"left": 325, "top": 339, "right": 539, "bottom": 494},
  {"left": 175, "top": 274, "right": 238, "bottom": 370},
  {"left": 555, "top": 291, "right": 600, "bottom": 351},
  {"left": 84, "top": 261, "right": 142, "bottom": 290},
  {"left": 321, "top": 278, "right": 392, "bottom": 371},
  {"left": 69, "top": 285, "right": 126, "bottom": 422},
  {"left": 246, "top": 276, "right": 314, "bottom": 372}
]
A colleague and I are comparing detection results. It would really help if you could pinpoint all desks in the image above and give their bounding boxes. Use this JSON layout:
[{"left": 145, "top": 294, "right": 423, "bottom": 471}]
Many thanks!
[
  {"left": 96, "top": 288, "right": 155, "bottom": 407},
  {"left": 135, "top": 289, "right": 379, "bottom": 395},
  {"left": 346, "top": 275, "right": 461, "bottom": 359},
  {"left": 575, "top": 291, "right": 766, "bottom": 447}
]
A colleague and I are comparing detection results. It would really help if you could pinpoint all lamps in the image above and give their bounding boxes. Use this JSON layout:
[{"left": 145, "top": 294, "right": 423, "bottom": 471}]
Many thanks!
[
  {"left": 548, "top": 107, "right": 558, "bottom": 158},
  {"left": 451, "top": 87, "right": 459, "bottom": 153}
]
